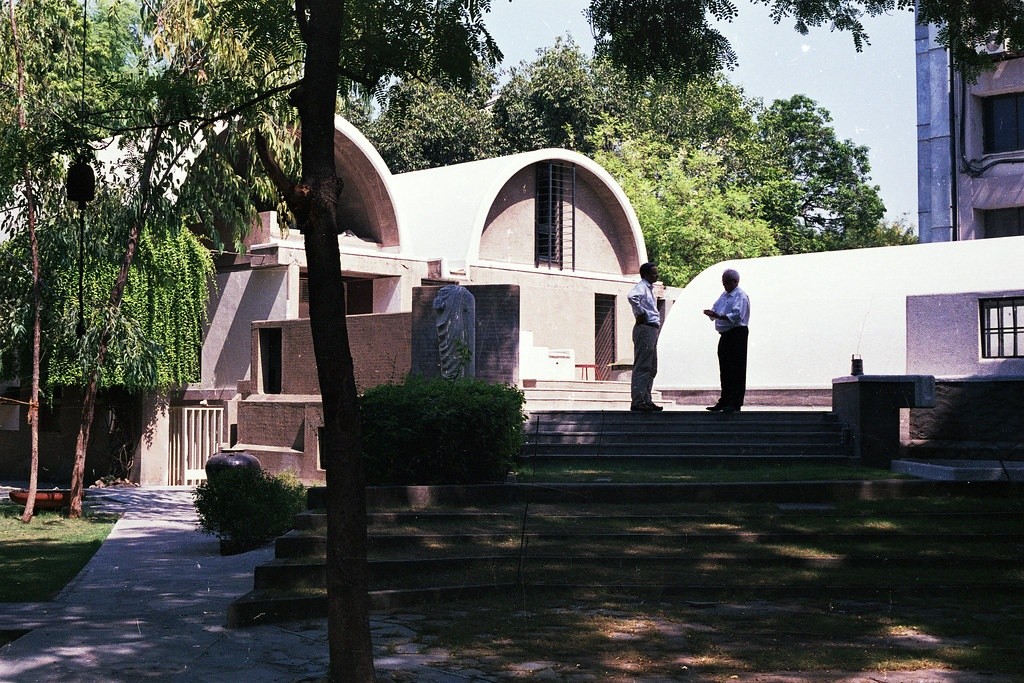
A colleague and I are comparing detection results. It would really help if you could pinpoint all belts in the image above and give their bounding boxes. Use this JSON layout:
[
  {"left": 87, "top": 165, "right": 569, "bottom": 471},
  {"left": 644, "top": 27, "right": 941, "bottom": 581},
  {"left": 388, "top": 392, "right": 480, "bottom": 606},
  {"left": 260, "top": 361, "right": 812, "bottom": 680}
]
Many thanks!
[{"left": 636, "top": 321, "right": 659, "bottom": 329}]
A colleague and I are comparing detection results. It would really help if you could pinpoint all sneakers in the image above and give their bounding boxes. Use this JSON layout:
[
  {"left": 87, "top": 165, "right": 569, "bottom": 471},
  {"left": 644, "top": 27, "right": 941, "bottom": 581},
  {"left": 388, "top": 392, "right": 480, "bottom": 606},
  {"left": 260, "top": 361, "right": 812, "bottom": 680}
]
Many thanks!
[
  {"left": 631, "top": 404, "right": 653, "bottom": 411},
  {"left": 647, "top": 401, "right": 663, "bottom": 411}
]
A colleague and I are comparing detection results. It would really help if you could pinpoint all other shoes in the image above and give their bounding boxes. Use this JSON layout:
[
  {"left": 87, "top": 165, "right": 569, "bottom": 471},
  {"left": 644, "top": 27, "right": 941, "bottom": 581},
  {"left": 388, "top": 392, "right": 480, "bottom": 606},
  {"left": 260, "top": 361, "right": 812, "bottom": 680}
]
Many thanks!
[
  {"left": 707, "top": 403, "right": 725, "bottom": 411},
  {"left": 718, "top": 408, "right": 740, "bottom": 416}
]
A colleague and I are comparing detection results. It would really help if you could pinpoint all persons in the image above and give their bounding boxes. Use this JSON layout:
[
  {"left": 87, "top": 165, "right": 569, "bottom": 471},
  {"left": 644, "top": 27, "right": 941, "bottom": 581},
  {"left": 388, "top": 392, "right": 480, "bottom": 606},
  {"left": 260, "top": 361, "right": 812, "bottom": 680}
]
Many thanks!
[
  {"left": 703, "top": 269, "right": 750, "bottom": 411},
  {"left": 628, "top": 263, "right": 663, "bottom": 412}
]
